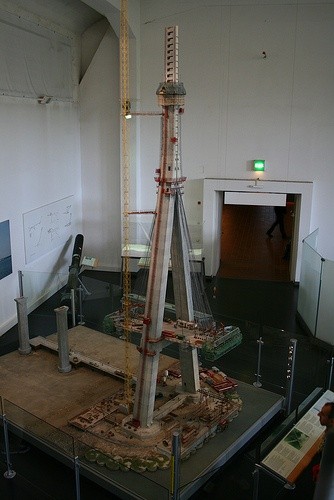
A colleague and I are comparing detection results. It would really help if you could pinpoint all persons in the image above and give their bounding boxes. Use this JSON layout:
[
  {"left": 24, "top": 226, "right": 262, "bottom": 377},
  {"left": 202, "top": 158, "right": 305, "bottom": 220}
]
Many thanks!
[{"left": 266, "top": 200, "right": 290, "bottom": 239}]
